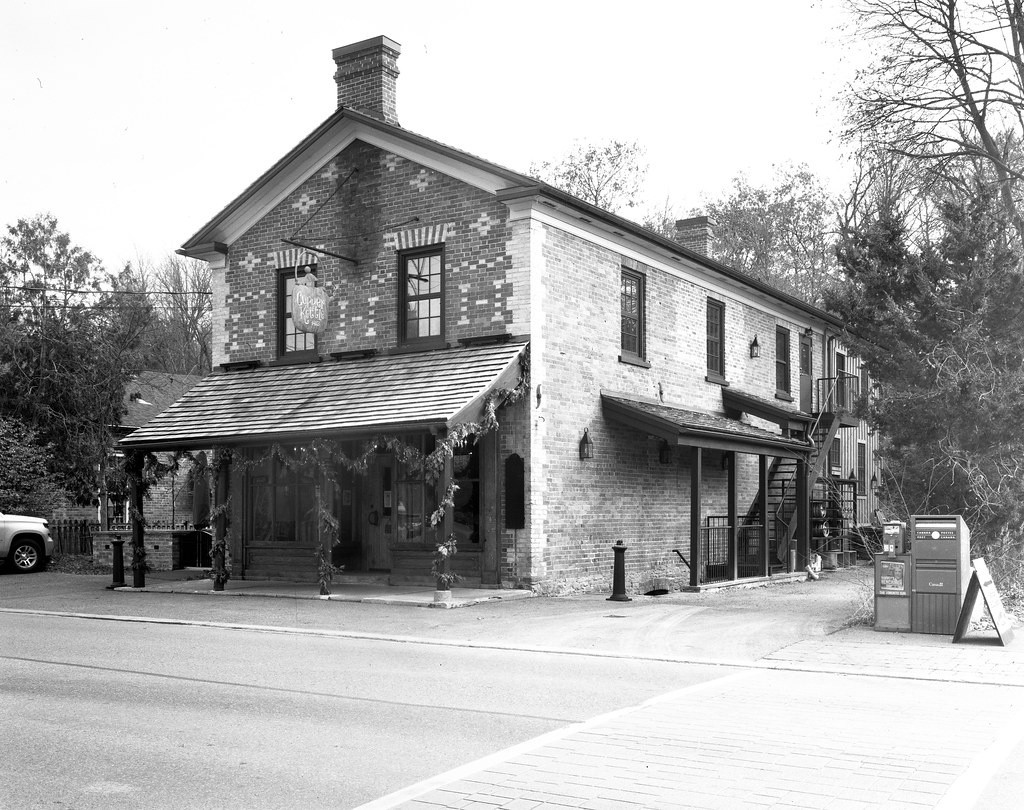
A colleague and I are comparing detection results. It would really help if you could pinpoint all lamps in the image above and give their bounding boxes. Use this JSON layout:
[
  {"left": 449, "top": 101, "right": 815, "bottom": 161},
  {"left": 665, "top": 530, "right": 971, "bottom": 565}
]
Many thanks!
[
  {"left": 807, "top": 327, "right": 813, "bottom": 335},
  {"left": 750, "top": 337, "right": 761, "bottom": 357},
  {"left": 579, "top": 427, "right": 593, "bottom": 459},
  {"left": 849, "top": 471, "right": 855, "bottom": 487},
  {"left": 871, "top": 471, "right": 877, "bottom": 489},
  {"left": 722, "top": 452, "right": 728, "bottom": 470},
  {"left": 660, "top": 440, "right": 672, "bottom": 464}
]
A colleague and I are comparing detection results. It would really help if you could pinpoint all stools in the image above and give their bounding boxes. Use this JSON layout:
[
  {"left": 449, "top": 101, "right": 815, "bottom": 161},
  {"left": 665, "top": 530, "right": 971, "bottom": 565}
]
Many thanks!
[
  {"left": 831, "top": 549, "right": 857, "bottom": 567},
  {"left": 820, "top": 552, "right": 844, "bottom": 569}
]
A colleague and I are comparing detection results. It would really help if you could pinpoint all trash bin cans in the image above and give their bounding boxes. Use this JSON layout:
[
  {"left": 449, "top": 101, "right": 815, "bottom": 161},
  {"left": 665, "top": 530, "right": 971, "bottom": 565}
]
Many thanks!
[
  {"left": 872, "top": 521, "right": 911, "bottom": 632},
  {"left": 911, "top": 511, "right": 973, "bottom": 637}
]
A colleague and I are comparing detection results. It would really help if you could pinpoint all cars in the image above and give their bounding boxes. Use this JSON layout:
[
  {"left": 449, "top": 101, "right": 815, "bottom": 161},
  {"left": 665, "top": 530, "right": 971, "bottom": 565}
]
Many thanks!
[{"left": 0, "top": 511, "right": 55, "bottom": 574}]
